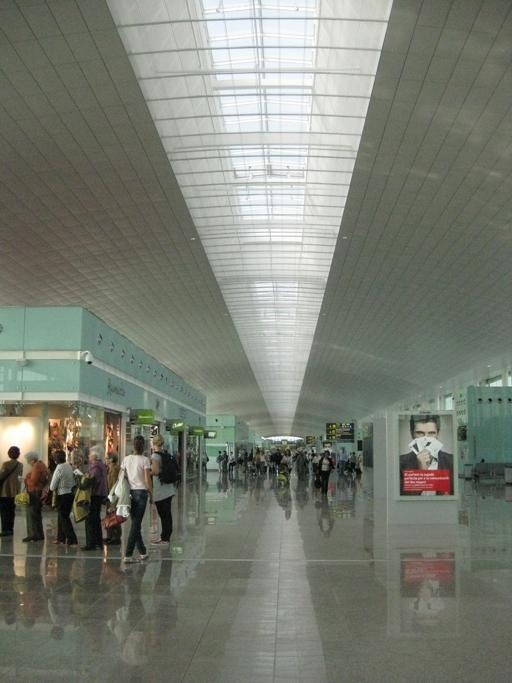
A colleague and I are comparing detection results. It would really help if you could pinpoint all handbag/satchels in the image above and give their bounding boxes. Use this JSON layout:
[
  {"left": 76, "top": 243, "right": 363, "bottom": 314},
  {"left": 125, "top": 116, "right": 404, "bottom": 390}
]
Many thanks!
[
  {"left": 101, "top": 512, "right": 128, "bottom": 530},
  {"left": 14, "top": 493, "right": 30, "bottom": 505},
  {"left": 314, "top": 479, "right": 322, "bottom": 488}
]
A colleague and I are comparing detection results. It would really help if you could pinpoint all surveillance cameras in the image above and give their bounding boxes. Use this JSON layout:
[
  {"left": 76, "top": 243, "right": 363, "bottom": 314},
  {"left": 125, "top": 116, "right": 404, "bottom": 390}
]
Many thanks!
[{"left": 86, "top": 354, "right": 93, "bottom": 365}]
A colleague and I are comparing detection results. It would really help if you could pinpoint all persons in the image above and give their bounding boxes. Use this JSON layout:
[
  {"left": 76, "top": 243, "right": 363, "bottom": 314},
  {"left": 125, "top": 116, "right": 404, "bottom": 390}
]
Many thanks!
[
  {"left": 0, "top": 446, "right": 23, "bottom": 538},
  {"left": 116, "top": 435, "right": 154, "bottom": 563},
  {"left": 21, "top": 451, "right": 51, "bottom": 543},
  {"left": 49, "top": 451, "right": 79, "bottom": 547},
  {"left": 73, "top": 444, "right": 109, "bottom": 550},
  {"left": 216, "top": 447, "right": 361, "bottom": 496},
  {"left": 102, "top": 453, "right": 124, "bottom": 545},
  {"left": 148, "top": 434, "right": 176, "bottom": 544},
  {"left": 398, "top": 415, "right": 454, "bottom": 495},
  {"left": 204, "top": 453, "right": 210, "bottom": 472}
]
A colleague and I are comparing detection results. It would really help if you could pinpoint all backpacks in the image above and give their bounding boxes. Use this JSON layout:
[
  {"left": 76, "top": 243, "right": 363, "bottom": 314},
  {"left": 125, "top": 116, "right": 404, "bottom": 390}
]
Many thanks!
[{"left": 158, "top": 450, "right": 178, "bottom": 483}]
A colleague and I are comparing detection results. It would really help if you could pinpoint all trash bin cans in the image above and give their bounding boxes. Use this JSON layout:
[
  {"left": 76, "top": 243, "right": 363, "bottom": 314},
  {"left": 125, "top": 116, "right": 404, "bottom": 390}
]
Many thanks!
[
  {"left": 464, "top": 464, "right": 473, "bottom": 480},
  {"left": 504, "top": 464, "right": 512, "bottom": 484}
]
